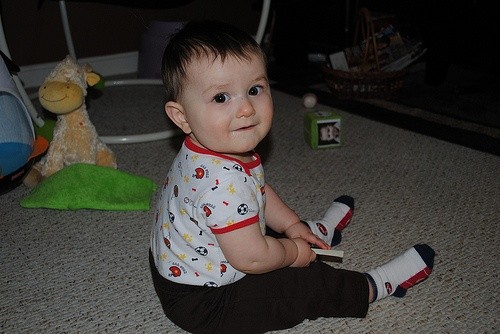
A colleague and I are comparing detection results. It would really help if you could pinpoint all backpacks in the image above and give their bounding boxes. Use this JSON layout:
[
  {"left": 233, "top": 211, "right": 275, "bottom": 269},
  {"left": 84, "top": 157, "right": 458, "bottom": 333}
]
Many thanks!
[{"left": 0, "top": 49, "right": 48, "bottom": 197}]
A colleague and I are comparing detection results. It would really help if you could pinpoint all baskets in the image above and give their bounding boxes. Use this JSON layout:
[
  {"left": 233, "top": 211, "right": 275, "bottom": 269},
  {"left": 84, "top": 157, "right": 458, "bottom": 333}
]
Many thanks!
[{"left": 320, "top": 6, "right": 419, "bottom": 98}]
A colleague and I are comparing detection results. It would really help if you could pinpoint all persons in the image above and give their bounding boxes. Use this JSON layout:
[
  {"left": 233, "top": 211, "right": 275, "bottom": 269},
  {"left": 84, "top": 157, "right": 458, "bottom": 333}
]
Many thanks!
[
  {"left": 147, "top": 21, "right": 436, "bottom": 334},
  {"left": 318, "top": 122, "right": 339, "bottom": 144}
]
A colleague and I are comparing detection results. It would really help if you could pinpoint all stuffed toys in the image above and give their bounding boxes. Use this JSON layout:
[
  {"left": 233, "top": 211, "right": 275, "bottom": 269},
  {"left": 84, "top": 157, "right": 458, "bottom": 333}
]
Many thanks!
[{"left": 22, "top": 54, "right": 117, "bottom": 188}]
